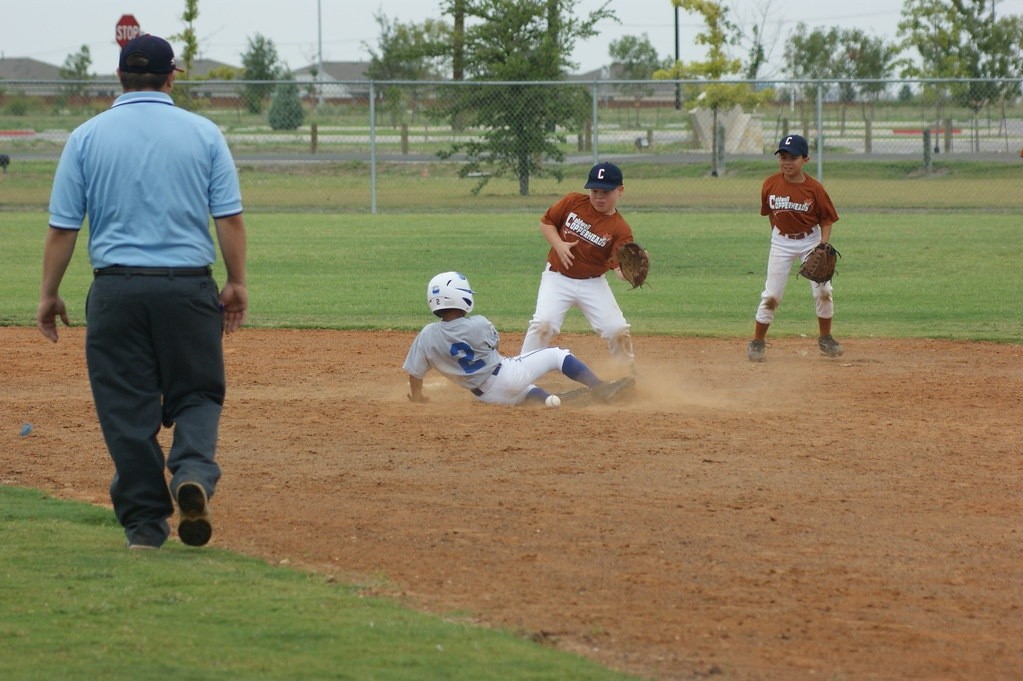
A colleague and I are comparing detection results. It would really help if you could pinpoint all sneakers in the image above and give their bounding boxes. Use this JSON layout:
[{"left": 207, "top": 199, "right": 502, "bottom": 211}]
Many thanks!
[
  {"left": 748, "top": 339, "right": 773, "bottom": 361},
  {"left": 600, "top": 375, "right": 636, "bottom": 405},
  {"left": 817, "top": 335, "right": 843, "bottom": 357},
  {"left": 557, "top": 387, "right": 592, "bottom": 408}
]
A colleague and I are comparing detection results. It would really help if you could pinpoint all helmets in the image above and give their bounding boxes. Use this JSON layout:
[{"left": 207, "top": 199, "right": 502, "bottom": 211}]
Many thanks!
[{"left": 428, "top": 271, "right": 475, "bottom": 317}]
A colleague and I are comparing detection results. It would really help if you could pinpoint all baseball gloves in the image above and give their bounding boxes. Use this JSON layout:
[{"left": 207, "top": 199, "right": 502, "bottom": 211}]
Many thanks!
[
  {"left": 795, "top": 242, "right": 843, "bottom": 288},
  {"left": 617, "top": 241, "right": 653, "bottom": 292}
]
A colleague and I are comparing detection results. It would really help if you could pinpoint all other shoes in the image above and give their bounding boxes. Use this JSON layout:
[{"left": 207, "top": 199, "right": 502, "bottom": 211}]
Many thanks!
[
  {"left": 176, "top": 481, "right": 213, "bottom": 546},
  {"left": 128, "top": 542, "right": 160, "bottom": 550}
]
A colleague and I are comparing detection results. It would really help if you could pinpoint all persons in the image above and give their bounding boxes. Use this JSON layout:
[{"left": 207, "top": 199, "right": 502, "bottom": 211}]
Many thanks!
[
  {"left": 747, "top": 135, "right": 842, "bottom": 362},
  {"left": 521, "top": 163, "right": 649, "bottom": 377},
  {"left": 401, "top": 271, "right": 635, "bottom": 407},
  {"left": 38, "top": 34, "right": 248, "bottom": 551}
]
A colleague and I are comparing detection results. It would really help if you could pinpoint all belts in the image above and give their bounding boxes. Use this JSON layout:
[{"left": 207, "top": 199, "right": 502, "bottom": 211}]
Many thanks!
[
  {"left": 470, "top": 362, "right": 502, "bottom": 397},
  {"left": 779, "top": 229, "right": 814, "bottom": 239},
  {"left": 549, "top": 266, "right": 601, "bottom": 279},
  {"left": 95, "top": 265, "right": 211, "bottom": 277}
]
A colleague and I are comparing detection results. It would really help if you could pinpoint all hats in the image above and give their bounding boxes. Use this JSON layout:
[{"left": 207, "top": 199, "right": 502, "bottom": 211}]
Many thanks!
[
  {"left": 774, "top": 135, "right": 809, "bottom": 157},
  {"left": 585, "top": 162, "right": 623, "bottom": 190},
  {"left": 119, "top": 33, "right": 185, "bottom": 75}
]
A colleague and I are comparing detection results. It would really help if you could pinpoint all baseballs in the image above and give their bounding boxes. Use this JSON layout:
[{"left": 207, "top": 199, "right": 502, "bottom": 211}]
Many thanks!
[{"left": 545, "top": 394, "right": 562, "bottom": 409}]
